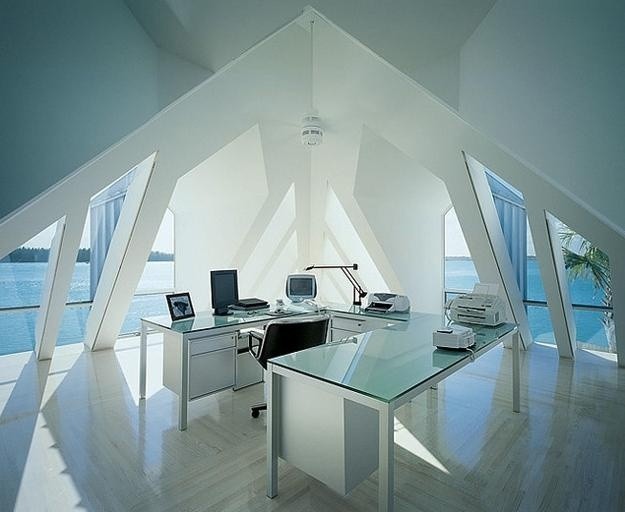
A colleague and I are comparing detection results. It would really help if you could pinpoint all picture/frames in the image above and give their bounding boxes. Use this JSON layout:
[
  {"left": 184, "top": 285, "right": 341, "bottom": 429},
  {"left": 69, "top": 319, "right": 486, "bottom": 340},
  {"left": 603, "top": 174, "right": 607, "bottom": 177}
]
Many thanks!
[{"left": 165, "top": 291, "right": 195, "bottom": 323}]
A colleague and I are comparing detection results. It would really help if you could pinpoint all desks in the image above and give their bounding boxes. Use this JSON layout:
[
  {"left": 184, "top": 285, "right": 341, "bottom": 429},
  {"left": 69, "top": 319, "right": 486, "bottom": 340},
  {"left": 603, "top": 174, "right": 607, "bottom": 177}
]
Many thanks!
[
  {"left": 266, "top": 313, "right": 521, "bottom": 511},
  {"left": 325, "top": 306, "right": 430, "bottom": 343},
  {"left": 140, "top": 302, "right": 325, "bottom": 435}
]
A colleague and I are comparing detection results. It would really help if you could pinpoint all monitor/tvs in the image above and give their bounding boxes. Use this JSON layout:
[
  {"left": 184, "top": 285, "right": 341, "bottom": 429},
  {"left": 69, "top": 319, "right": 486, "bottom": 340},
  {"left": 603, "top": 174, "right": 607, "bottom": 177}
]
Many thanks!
[
  {"left": 286, "top": 274, "right": 317, "bottom": 303},
  {"left": 211, "top": 269, "right": 239, "bottom": 316}
]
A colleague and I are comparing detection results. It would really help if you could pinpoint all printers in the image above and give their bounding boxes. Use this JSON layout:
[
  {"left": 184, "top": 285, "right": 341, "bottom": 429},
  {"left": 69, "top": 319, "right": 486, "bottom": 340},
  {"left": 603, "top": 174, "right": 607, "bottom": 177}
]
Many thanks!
[
  {"left": 365, "top": 292, "right": 410, "bottom": 314},
  {"left": 445, "top": 282, "right": 506, "bottom": 326}
]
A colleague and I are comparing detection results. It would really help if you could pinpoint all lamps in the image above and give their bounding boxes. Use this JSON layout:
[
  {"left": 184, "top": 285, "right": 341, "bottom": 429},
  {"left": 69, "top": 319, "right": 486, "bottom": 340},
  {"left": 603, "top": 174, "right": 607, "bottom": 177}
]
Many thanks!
[
  {"left": 305, "top": 263, "right": 368, "bottom": 307},
  {"left": 300, "top": 113, "right": 323, "bottom": 148}
]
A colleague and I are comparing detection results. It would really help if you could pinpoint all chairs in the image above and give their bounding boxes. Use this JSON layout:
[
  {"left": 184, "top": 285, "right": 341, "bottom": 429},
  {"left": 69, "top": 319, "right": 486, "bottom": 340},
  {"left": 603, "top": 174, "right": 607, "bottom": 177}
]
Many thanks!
[{"left": 248, "top": 314, "right": 330, "bottom": 418}]
